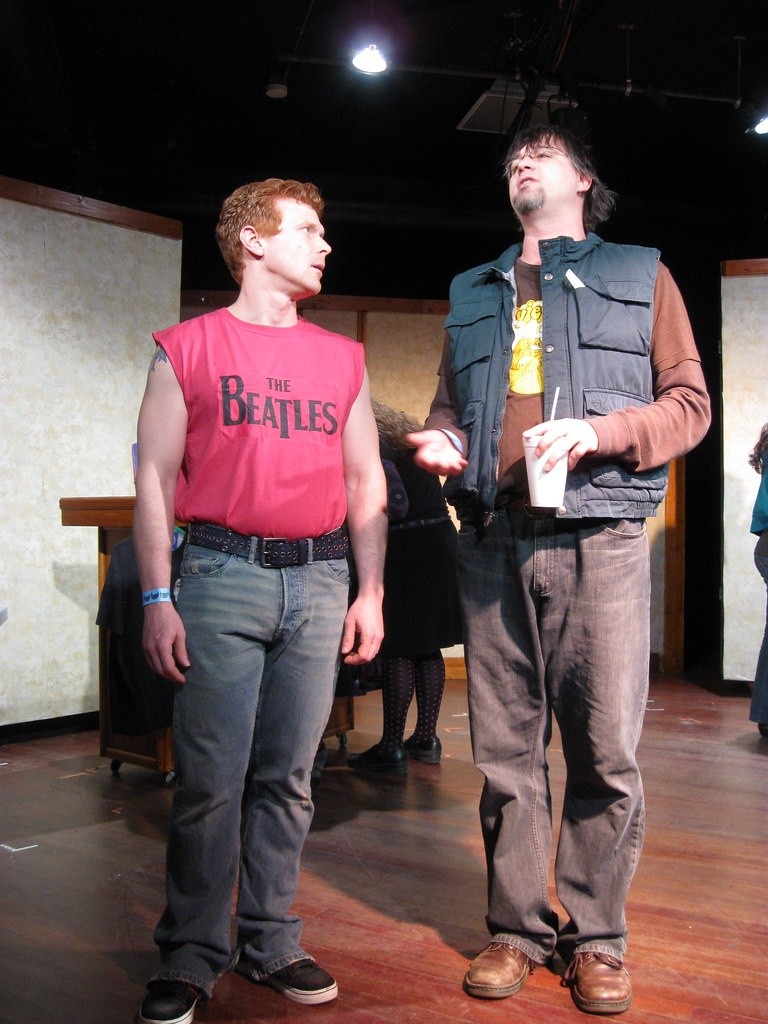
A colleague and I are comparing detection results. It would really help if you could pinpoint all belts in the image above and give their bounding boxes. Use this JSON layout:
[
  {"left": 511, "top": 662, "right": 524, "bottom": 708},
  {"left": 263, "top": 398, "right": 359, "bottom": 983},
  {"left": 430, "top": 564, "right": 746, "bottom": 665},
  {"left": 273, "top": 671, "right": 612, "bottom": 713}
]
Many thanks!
[{"left": 186, "top": 520, "right": 349, "bottom": 569}]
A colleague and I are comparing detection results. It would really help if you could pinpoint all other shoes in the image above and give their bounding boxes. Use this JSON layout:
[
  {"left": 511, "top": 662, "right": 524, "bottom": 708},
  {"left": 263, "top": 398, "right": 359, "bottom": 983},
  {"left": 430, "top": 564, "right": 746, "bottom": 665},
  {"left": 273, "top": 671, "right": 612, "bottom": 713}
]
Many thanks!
[
  {"left": 346, "top": 743, "right": 408, "bottom": 776},
  {"left": 402, "top": 735, "right": 442, "bottom": 764}
]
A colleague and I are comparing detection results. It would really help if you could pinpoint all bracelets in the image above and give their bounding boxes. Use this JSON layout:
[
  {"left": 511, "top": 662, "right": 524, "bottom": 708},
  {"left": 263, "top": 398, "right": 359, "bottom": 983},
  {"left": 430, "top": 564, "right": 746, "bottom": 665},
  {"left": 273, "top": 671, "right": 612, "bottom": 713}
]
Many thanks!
[{"left": 142, "top": 588, "right": 171, "bottom": 607}]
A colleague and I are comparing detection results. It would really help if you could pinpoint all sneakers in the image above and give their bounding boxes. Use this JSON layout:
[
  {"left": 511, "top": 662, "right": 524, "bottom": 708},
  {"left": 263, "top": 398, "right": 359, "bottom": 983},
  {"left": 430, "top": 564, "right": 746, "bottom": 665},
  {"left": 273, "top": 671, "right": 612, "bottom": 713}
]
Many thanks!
[
  {"left": 137, "top": 980, "right": 199, "bottom": 1024},
  {"left": 267, "top": 957, "right": 338, "bottom": 1004}
]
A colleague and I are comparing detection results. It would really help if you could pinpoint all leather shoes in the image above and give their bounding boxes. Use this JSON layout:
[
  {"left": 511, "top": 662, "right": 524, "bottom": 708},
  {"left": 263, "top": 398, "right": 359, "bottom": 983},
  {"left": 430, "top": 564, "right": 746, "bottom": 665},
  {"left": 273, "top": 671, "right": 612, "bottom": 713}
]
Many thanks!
[
  {"left": 505, "top": 492, "right": 556, "bottom": 520},
  {"left": 563, "top": 952, "right": 632, "bottom": 1013},
  {"left": 462, "top": 942, "right": 533, "bottom": 998}
]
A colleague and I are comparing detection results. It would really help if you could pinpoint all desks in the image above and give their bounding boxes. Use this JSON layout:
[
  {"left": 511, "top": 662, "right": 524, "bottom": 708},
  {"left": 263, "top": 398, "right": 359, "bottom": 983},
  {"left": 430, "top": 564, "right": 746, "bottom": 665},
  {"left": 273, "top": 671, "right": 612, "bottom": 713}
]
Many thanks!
[{"left": 59, "top": 496, "right": 354, "bottom": 785}]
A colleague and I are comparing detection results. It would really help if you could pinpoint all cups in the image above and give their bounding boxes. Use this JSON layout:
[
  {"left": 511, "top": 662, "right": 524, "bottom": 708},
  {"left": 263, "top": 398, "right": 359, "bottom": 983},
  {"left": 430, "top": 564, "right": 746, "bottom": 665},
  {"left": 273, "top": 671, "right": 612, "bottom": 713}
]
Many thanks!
[{"left": 522, "top": 436, "right": 567, "bottom": 508}]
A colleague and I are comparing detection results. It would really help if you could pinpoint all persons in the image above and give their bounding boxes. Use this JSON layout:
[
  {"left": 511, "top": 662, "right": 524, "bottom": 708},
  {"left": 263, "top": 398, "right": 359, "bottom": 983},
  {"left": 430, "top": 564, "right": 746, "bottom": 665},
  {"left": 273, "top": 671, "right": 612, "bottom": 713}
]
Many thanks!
[
  {"left": 131, "top": 178, "right": 388, "bottom": 1023},
  {"left": 348, "top": 396, "right": 463, "bottom": 768},
  {"left": 748, "top": 423, "right": 768, "bottom": 737},
  {"left": 408, "top": 125, "right": 712, "bottom": 1014}
]
